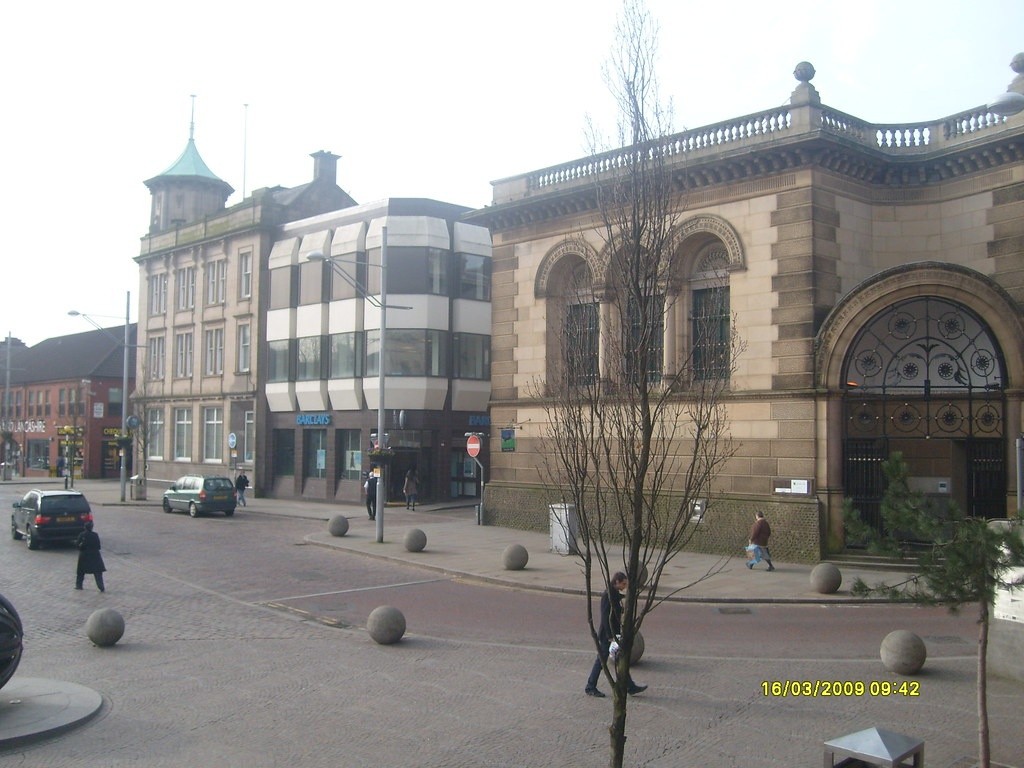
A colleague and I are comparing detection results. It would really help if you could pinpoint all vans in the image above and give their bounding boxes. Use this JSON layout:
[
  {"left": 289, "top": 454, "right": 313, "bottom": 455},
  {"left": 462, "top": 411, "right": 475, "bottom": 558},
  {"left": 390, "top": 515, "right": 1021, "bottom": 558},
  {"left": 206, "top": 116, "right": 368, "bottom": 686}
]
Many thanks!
[{"left": 163, "top": 474, "right": 237, "bottom": 518}]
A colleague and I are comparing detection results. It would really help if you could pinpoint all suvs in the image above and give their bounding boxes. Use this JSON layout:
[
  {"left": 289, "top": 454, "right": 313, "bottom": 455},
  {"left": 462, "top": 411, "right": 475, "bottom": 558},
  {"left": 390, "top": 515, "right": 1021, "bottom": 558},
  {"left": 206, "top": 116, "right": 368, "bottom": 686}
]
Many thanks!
[{"left": 11, "top": 489, "right": 93, "bottom": 549}]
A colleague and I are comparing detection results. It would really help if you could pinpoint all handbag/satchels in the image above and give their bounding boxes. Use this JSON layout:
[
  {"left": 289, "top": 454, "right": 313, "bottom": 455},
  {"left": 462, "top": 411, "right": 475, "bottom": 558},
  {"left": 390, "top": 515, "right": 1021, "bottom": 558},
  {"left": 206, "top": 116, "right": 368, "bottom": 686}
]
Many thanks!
[
  {"left": 745, "top": 544, "right": 760, "bottom": 563},
  {"left": 78, "top": 532, "right": 87, "bottom": 550}
]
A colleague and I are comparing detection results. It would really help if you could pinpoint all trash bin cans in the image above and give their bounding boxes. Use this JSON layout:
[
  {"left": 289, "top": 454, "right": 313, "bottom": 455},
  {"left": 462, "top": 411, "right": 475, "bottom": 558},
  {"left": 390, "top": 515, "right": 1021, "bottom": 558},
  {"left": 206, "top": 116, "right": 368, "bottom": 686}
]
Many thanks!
[
  {"left": 1, "top": 462, "right": 13, "bottom": 480},
  {"left": 550, "top": 504, "right": 578, "bottom": 554},
  {"left": 129, "top": 474, "right": 147, "bottom": 501}
]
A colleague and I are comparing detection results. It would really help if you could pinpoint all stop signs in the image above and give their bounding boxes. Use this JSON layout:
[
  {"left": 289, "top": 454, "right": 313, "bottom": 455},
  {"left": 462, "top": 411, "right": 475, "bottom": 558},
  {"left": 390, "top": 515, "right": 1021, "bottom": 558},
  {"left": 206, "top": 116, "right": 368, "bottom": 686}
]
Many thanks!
[{"left": 467, "top": 436, "right": 481, "bottom": 457}]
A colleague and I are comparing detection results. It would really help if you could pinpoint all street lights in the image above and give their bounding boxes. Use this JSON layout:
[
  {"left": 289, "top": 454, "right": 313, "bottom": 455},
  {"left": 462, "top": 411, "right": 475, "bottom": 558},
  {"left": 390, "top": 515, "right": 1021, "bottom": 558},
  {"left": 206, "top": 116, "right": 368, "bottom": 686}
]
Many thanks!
[
  {"left": 306, "top": 227, "right": 412, "bottom": 542},
  {"left": 68, "top": 290, "right": 147, "bottom": 502}
]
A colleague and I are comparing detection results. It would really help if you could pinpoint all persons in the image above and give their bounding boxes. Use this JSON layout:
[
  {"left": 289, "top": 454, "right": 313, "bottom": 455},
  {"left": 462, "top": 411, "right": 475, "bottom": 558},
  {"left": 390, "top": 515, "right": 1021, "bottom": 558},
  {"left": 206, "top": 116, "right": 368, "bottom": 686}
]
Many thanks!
[
  {"left": 585, "top": 571, "right": 648, "bottom": 697},
  {"left": 745, "top": 511, "right": 774, "bottom": 571},
  {"left": 363, "top": 471, "right": 377, "bottom": 520},
  {"left": 235, "top": 470, "right": 249, "bottom": 507},
  {"left": 72, "top": 521, "right": 108, "bottom": 592},
  {"left": 403, "top": 464, "right": 419, "bottom": 511}
]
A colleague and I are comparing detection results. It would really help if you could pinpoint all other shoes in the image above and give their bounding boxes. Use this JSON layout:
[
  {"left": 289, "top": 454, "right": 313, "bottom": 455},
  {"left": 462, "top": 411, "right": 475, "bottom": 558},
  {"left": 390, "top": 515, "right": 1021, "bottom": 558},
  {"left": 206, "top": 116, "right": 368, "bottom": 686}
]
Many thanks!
[
  {"left": 628, "top": 685, "right": 647, "bottom": 695},
  {"left": 586, "top": 688, "right": 605, "bottom": 697},
  {"left": 368, "top": 515, "right": 375, "bottom": 520},
  {"left": 407, "top": 507, "right": 409, "bottom": 509},
  {"left": 746, "top": 562, "right": 753, "bottom": 569},
  {"left": 413, "top": 509, "right": 415, "bottom": 511},
  {"left": 766, "top": 566, "right": 774, "bottom": 571}
]
